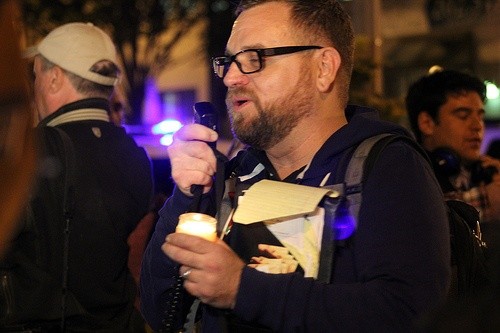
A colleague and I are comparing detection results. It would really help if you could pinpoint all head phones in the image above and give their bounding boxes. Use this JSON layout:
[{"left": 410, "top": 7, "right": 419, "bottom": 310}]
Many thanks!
[{"left": 425, "top": 147, "right": 494, "bottom": 186}]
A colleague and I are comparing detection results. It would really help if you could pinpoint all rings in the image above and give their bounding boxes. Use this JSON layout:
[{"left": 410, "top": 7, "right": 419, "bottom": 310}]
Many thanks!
[{"left": 183, "top": 268, "right": 197, "bottom": 280}]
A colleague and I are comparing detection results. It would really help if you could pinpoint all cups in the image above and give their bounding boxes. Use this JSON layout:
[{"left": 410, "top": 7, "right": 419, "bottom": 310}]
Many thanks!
[{"left": 175, "top": 212, "right": 219, "bottom": 243}]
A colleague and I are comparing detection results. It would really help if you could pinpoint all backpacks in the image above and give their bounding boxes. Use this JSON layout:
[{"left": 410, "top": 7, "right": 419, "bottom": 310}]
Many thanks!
[{"left": 329, "top": 130, "right": 488, "bottom": 298}]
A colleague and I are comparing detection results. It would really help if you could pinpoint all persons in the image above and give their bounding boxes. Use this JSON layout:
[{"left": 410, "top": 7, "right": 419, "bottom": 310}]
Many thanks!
[
  {"left": 139, "top": 0, "right": 451, "bottom": 333},
  {"left": 405, "top": 69, "right": 500, "bottom": 333},
  {"left": 0, "top": 0, "right": 156, "bottom": 333}
]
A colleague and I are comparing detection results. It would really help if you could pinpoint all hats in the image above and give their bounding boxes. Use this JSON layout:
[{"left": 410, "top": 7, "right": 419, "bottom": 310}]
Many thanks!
[{"left": 22, "top": 22, "right": 124, "bottom": 86}]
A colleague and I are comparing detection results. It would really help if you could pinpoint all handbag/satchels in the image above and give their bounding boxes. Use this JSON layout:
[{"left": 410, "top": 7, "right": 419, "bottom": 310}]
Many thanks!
[{"left": 0, "top": 126, "right": 88, "bottom": 324}]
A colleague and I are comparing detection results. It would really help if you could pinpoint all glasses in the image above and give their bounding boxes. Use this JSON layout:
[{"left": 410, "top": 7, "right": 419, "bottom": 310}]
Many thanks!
[{"left": 210, "top": 45, "right": 325, "bottom": 79}]
